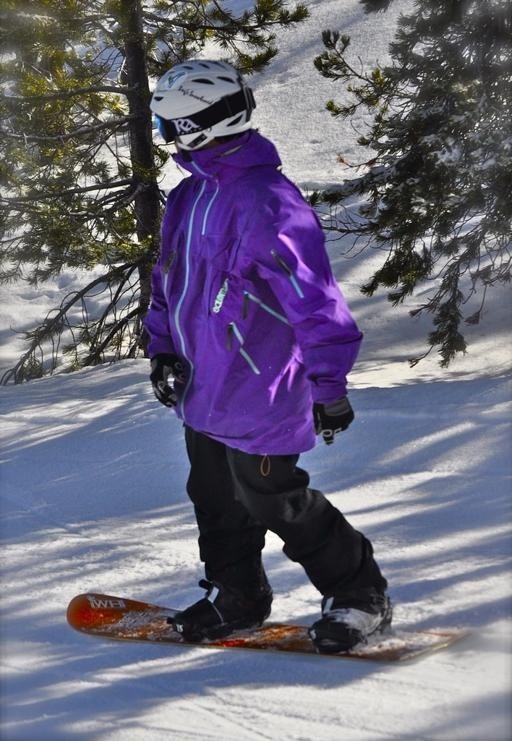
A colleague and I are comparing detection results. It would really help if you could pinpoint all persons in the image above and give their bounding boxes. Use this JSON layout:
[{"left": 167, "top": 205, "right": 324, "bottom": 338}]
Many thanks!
[{"left": 140, "top": 62, "right": 392, "bottom": 655}]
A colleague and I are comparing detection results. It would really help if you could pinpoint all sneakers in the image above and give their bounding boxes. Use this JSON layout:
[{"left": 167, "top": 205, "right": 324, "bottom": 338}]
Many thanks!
[
  {"left": 307, "top": 587, "right": 393, "bottom": 653},
  {"left": 171, "top": 577, "right": 275, "bottom": 642}
]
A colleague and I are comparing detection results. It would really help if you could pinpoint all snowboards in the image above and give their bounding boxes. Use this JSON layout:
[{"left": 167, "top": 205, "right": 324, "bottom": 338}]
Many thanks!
[{"left": 66, "top": 592, "right": 469, "bottom": 662}]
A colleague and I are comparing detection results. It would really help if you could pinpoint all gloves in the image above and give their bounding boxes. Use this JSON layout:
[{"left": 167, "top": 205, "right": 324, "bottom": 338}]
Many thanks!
[
  {"left": 148, "top": 351, "right": 189, "bottom": 408},
  {"left": 311, "top": 395, "right": 355, "bottom": 447}
]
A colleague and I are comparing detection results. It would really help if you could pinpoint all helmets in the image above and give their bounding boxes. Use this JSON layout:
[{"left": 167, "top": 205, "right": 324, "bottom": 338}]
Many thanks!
[{"left": 149, "top": 59, "right": 257, "bottom": 152}]
